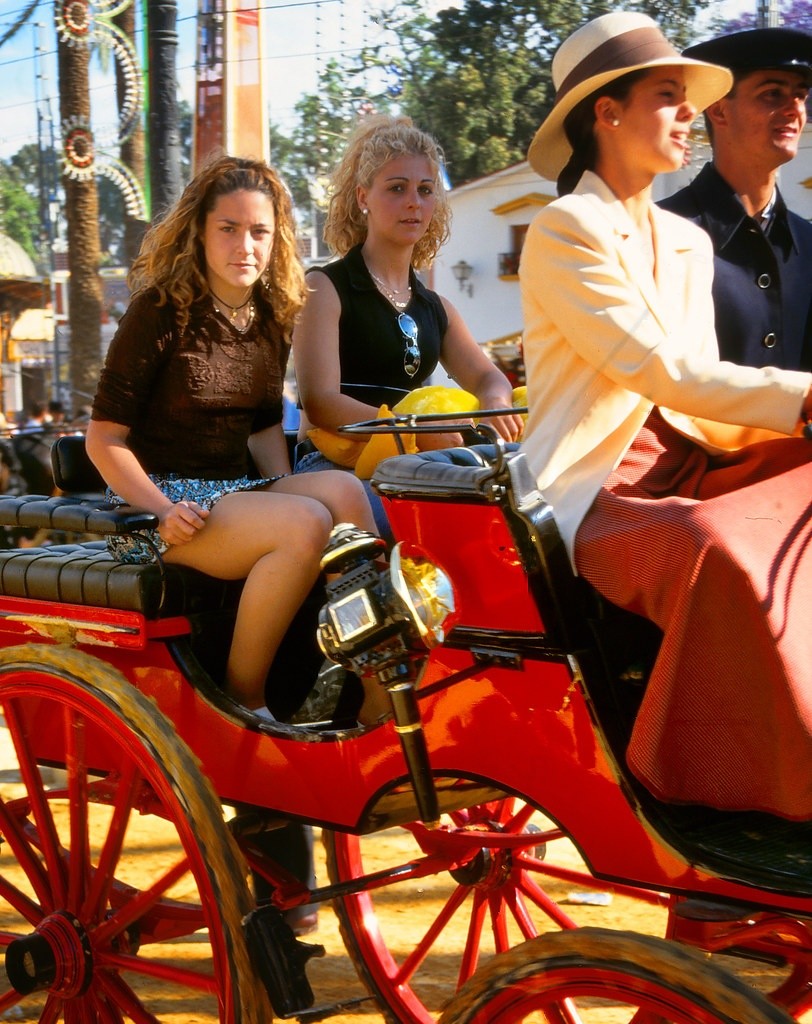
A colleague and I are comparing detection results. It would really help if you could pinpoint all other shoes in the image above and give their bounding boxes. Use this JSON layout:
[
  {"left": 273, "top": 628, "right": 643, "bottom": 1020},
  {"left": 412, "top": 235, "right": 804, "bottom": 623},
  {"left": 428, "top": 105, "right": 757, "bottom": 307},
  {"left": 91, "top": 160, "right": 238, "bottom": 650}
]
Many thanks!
[{"left": 285, "top": 911, "right": 318, "bottom": 933}]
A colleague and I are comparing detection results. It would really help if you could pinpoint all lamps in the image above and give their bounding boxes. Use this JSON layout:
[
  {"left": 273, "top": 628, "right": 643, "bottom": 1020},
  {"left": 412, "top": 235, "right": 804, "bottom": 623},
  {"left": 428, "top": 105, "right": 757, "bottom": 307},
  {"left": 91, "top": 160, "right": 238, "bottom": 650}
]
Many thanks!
[{"left": 452, "top": 259, "right": 476, "bottom": 302}]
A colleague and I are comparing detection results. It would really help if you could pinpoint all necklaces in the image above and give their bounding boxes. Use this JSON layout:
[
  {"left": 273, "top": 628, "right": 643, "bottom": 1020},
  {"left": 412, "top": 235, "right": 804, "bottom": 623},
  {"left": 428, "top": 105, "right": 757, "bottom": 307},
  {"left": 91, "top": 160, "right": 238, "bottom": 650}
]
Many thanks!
[
  {"left": 209, "top": 288, "right": 254, "bottom": 332},
  {"left": 369, "top": 269, "right": 412, "bottom": 307}
]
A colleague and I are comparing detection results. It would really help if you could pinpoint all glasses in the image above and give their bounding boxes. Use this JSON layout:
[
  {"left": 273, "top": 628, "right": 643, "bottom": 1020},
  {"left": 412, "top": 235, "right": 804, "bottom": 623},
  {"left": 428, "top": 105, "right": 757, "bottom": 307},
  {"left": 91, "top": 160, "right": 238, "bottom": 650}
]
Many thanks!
[{"left": 398, "top": 310, "right": 421, "bottom": 378}]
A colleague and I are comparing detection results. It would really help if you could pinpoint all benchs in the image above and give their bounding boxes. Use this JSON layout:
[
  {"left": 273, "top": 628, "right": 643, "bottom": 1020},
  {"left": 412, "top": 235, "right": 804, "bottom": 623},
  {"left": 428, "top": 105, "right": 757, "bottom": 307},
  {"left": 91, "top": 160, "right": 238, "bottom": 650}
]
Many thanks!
[
  {"left": 371, "top": 440, "right": 576, "bottom": 644},
  {"left": 1, "top": 429, "right": 337, "bottom": 615}
]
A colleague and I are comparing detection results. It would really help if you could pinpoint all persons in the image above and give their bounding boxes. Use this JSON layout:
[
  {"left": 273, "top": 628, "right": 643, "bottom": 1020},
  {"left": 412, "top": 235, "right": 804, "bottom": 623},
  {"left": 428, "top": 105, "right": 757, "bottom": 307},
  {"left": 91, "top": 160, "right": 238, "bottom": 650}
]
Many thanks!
[
  {"left": 292, "top": 113, "right": 525, "bottom": 547},
  {"left": 517, "top": 12, "right": 812, "bottom": 819},
  {"left": 85, "top": 155, "right": 395, "bottom": 729},
  {"left": 655, "top": 27, "right": 812, "bottom": 372},
  {"left": 0, "top": 401, "right": 93, "bottom": 439}
]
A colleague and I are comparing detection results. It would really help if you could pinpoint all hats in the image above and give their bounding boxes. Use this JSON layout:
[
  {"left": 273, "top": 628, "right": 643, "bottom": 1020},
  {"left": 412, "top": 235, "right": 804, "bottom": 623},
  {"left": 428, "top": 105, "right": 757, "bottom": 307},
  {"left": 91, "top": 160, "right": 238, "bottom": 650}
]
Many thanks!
[
  {"left": 680, "top": 27, "right": 812, "bottom": 74},
  {"left": 529, "top": 12, "right": 731, "bottom": 182},
  {"left": 47, "top": 400, "right": 71, "bottom": 413}
]
